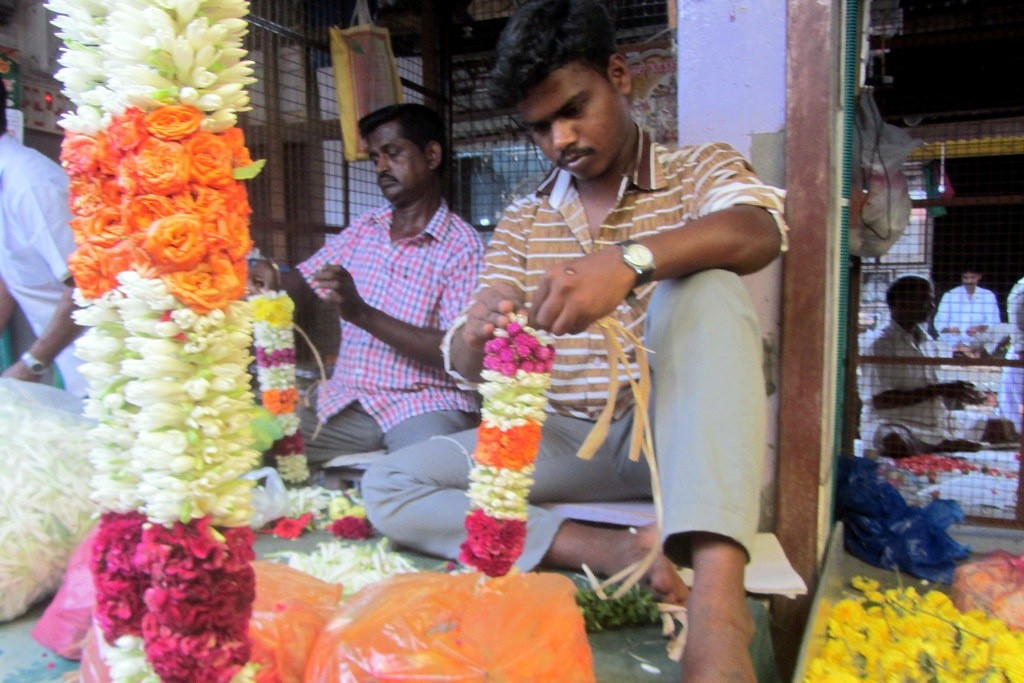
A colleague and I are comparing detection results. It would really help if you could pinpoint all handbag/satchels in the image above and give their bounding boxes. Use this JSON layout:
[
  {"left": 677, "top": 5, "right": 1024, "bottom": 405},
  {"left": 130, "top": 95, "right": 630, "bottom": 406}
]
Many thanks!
[{"left": 331, "top": 0, "right": 404, "bottom": 163}]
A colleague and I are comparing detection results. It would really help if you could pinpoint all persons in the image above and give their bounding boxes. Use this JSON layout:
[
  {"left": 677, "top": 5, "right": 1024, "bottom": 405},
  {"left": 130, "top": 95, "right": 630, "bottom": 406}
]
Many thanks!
[
  {"left": 363, "top": 4, "right": 790, "bottom": 683},
  {"left": 247, "top": 102, "right": 488, "bottom": 492},
  {"left": 0, "top": 80, "right": 91, "bottom": 400},
  {"left": 857, "top": 257, "right": 1024, "bottom": 461}
]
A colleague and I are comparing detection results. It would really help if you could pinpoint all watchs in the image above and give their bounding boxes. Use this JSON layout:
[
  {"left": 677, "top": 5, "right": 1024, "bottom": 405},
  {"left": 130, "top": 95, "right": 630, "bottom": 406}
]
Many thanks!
[
  {"left": 19, "top": 351, "right": 53, "bottom": 377},
  {"left": 613, "top": 240, "right": 657, "bottom": 289}
]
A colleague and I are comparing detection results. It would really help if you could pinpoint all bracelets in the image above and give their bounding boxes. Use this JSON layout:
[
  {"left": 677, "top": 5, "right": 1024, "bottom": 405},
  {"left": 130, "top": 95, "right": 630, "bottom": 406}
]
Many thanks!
[{"left": 266, "top": 258, "right": 281, "bottom": 290}]
